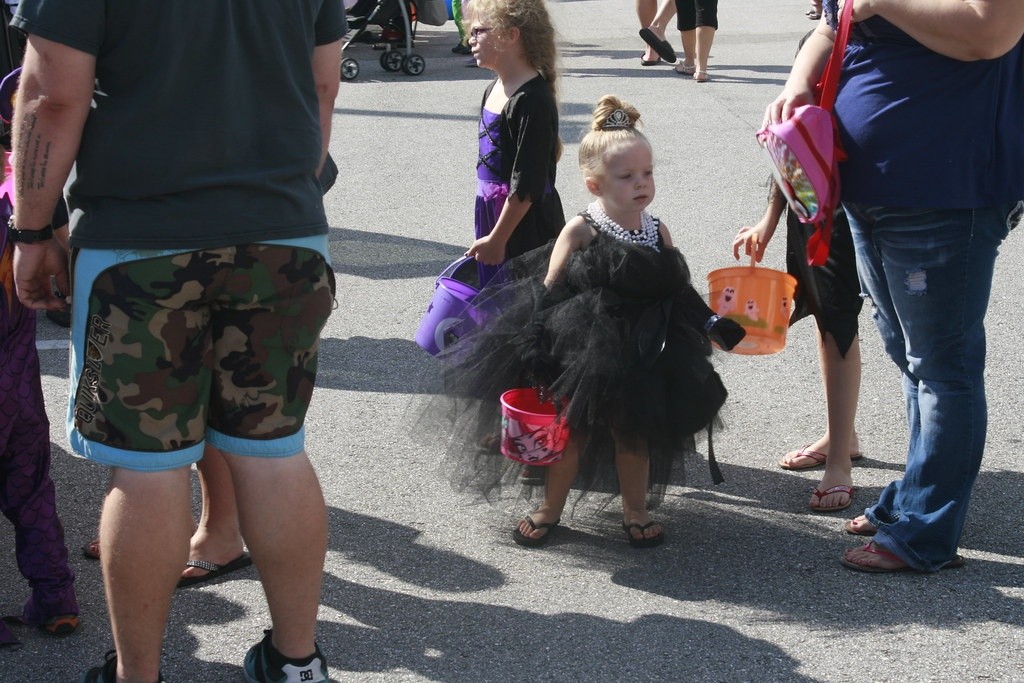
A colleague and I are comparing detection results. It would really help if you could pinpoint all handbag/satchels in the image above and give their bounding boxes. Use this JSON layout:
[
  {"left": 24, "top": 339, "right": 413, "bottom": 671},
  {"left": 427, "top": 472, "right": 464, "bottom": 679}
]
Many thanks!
[{"left": 756, "top": 104, "right": 848, "bottom": 265}]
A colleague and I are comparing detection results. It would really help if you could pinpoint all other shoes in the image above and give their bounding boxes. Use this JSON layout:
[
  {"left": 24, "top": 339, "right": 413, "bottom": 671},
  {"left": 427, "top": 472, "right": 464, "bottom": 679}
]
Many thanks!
[
  {"left": 464, "top": 59, "right": 477, "bottom": 68},
  {"left": 452, "top": 44, "right": 471, "bottom": 54}
]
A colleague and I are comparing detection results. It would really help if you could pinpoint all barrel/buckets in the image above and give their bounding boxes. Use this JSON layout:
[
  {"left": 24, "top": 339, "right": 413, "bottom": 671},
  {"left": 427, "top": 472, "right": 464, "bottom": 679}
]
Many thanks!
[
  {"left": 499, "top": 387, "right": 570, "bottom": 466},
  {"left": 414, "top": 253, "right": 495, "bottom": 364},
  {"left": 707, "top": 232, "right": 799, "bottom": 357}
]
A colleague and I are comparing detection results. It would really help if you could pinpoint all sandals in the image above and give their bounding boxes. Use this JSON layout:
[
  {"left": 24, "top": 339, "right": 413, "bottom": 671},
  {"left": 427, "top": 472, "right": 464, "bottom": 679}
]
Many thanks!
[
  {"left": 675, "top": 61, "right": 696, "bottom": 74},
  {"left": 692, "top": 70, "right": 711, "bottom": 82}
]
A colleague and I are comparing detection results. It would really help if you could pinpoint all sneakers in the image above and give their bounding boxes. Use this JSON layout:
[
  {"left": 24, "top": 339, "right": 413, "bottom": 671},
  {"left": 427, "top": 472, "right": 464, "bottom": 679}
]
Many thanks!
[
  {"left": 81, "top": 650, "right": 167, "bottom": 683},
  {"left": 24, "top": 601, "right": 78, "bottom": 635},
  {"left": 243, "top": 626, "right": 329, "bottom": 683}
]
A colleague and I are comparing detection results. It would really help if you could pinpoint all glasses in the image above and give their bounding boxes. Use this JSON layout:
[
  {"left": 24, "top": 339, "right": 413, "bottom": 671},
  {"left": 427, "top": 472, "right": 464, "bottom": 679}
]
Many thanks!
[{"left": 471, "top": 26, "right": 509, "bottom": 38}]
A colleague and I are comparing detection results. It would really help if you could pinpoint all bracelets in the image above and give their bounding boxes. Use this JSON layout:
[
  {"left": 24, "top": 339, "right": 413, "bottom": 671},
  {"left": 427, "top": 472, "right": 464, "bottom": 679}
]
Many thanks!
[
  {"left": 703, "top": 315, "right": 722, "bottom": 335},
  {"left": 7, "top": 215, "right": 52, "bottom": 244}
]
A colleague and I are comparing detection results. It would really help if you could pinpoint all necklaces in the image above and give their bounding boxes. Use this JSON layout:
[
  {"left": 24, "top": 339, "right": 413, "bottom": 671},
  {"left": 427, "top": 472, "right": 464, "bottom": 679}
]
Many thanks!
[{"left": 586, "top": 198, "right": 661, "bottom": 253}]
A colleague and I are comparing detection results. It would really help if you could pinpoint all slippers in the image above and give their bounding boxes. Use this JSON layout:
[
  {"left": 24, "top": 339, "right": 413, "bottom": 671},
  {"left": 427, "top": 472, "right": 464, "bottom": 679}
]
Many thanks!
[
  {"left": 809, "top": 485, "right": 854, "bottom": 512},
  {"left": 84, "top": 540, "right": 99, "bottom": 560},
  {"left": 622, "top": 519, "right": 665, "bottom": 549},
  {"left": 639, "top": 28, "right": 677, "bottom": 63},
  {"left": 841, "top": 541, "right": 915, "bottom": 572},
  {"left": 177, "top": 551, "right": 252, "bottom": 587},
  {"left": 842, "top": 515, "right": 878, "bottom": 536},
  {"left": 777, "top": 442, "right": 862, "bottom": 470},
  {"left": 512, "top": 515, "right": 561, "bottom": 547},
  {"left": 640, "top": 53, "right": 661, "bottom": 66}
]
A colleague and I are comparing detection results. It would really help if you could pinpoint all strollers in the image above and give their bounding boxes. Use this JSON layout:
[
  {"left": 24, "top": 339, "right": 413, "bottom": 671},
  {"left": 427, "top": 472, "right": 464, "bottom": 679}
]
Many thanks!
[
  {"left": 337, "top": 0, "right": 427, "bottom": 82},
  {"left": 0, "top": 66, "right": 22, "bottom": 253}
]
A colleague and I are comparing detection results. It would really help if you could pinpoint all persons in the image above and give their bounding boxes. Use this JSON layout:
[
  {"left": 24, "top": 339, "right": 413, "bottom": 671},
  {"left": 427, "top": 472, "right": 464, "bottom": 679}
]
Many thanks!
[
  {"left": 734, "top": 29, "right": 865, "bottom": 510},
  {"left": 675, "top": 0, "right": 718, "bottom": 81},
  {"left": 761, "top": 0, "right": 1024, "bottom": 575},
  {"left": 635, "top": 0, "right": 677, "bottom": 66},
  {"left": 510, "top": 95, "right": 747, "bottom": 551},
  {"left": 452, "top": 0, "right": 478, "bottom": 67},
  {"left": 461, "top": 0, "right": 566, "bottom": 451},
  {"left": 7, "top": 0, "right": 348, "bottom": 683},
  {"left": 0, "top": 0, "right": 254, "bottom": 635}
]
